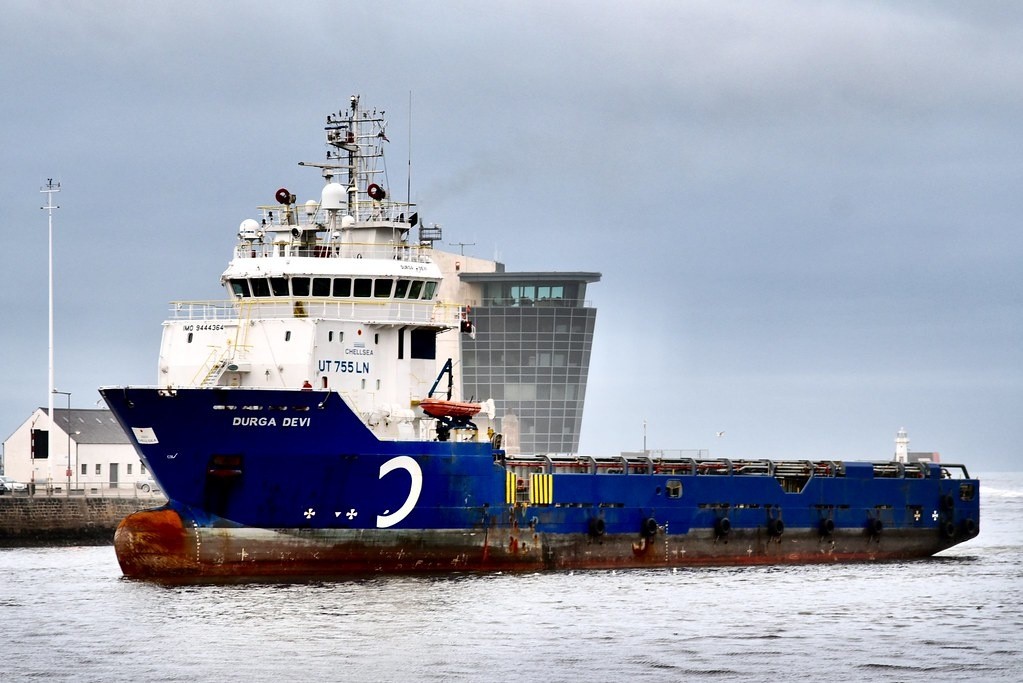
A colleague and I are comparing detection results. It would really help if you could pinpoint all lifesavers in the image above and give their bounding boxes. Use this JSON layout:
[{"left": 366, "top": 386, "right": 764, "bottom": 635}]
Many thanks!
[
  {"left": 767, "top": 519, "right": 784, "bottom": 536},
  {"left": 961, "top": 519, "right": 976, "bottom": 534},
  {"left": 867, "top": 518, "right": 883, "bottom": 537},
  {"left": 589, "top": 518, "right": 605, "bottom": 537},
  {"left": 642, "top": 517, "right": 657, "bottom": 537},
  {"left": 714, "top": 516, "right": 731, "bottom": 536},
  {"left": 817, "top": 518, "right": 834, "bottom": 537},
  {"left": 939, "top": 522, "right": 956, "bottom": 537}
]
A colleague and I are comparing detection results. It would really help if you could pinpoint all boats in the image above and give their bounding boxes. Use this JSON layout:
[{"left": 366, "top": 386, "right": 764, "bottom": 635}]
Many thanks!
[
  {"left": 419, "top": 397, "right": 483, "bottom": 417},
  {"left": 94, "top": 87, "right": 982, "bottom": 588}
]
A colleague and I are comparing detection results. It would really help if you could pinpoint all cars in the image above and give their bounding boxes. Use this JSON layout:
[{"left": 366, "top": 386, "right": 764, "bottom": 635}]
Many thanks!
[
  {"left": 136, "top": 476, "right": 161, "bottom": 492},
  {"left": 0, "top": 475, "right": 25, "bottom": 495}
]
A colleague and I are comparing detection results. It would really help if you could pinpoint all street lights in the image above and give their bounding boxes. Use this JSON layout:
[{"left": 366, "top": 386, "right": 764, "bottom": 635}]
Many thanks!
[
  {"left": 52, "top": 389, "right": 81, "bottom": 496},
  {"left": 38, "top": 178, "right": 61, "bottom": 494}
]
